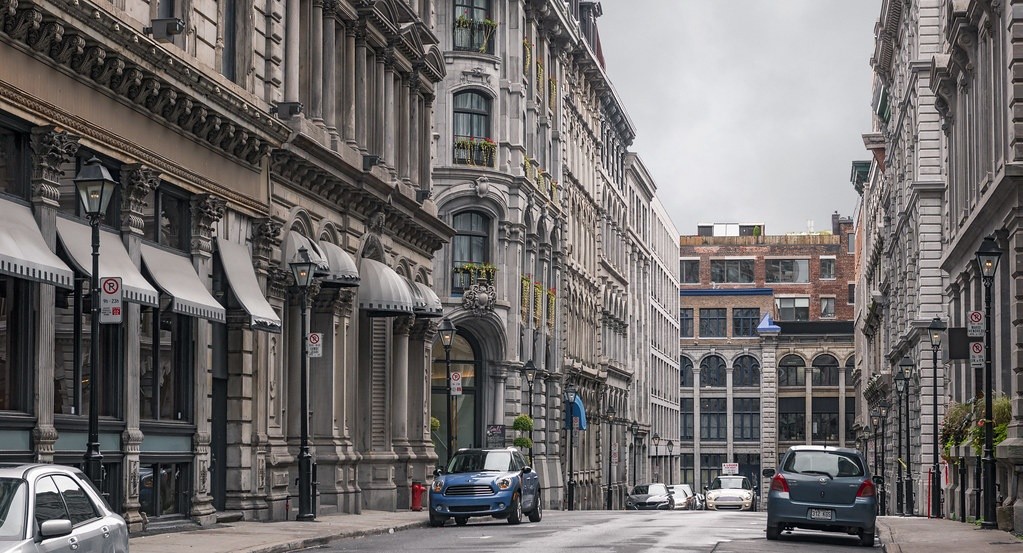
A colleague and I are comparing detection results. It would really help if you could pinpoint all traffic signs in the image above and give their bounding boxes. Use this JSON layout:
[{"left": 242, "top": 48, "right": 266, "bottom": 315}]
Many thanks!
[{"left": 970, "top": 341, "right": 985, "bottom": 368}]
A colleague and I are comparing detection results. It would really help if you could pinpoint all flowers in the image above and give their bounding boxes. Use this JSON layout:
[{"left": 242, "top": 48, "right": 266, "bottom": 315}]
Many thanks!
[
  {"left": 521, "top": 275, "right": 557, "bottom": 337},
  {"left": 454, "top": 136, "right": 497, "bottom": 170}
]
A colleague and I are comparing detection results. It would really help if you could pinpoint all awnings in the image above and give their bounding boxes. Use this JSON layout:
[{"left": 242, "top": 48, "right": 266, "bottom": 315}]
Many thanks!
[
  {"left": 140, "top": 244, "right": 225, "bottom": 323},
  {"left": 284, "top": 230, "right": 360, "bottom": 287},
  {"left": 217, "top": 238, "right": 281, "bottom": 334},
  {"left": 56, "top": 217, "right": 158, "bottom": 308},
  {"left": 358, "top": 258, "right": 444, "bottom": 317},
  {"left": 0, "top": 198, "right": 73, "bottom": 290}
]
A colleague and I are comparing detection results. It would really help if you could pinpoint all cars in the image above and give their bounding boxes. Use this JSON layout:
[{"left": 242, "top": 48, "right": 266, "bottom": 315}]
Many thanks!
[
  {"left": 761, "top": 445, "right": 884, "bottom": 547},
  {"left": 624, "top": 482, "right": 675, "bottom": 510},
  {"left": 667, "top": 484, "right": 706, "bottom": 510},
  {"left": 704, "top": 476, "right": 758, "bottom": 511},
  {"left": 0, "top": 461, "right": 129, "bottom": 553},
  {"left": 429, "top": 446, "right": 542, "bottom": 527}
]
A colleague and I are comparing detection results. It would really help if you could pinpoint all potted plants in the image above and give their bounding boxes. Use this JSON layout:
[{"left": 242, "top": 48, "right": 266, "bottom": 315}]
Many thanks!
[
  {"left": 455, "top": 16, "right": 497, "bottom": 53},
  {"left": 454, "top": 263, "right": 500, "bottom": 293}
]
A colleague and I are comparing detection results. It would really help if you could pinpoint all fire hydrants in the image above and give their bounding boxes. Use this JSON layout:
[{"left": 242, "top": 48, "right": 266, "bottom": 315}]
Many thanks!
[{"left": 411, "top": 481, "right": 426, "bottom": 511}]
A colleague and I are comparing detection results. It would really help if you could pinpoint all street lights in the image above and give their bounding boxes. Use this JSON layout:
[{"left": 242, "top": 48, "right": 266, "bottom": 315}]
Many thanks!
[
  {"left": 863, "top": 425, "right": 871, "bottom": 463},
  {"left": 564, "top": 382, "right": 578, "bottom": 511},
  {"left": 288, "top": 243, "right": 318, "bottom": 521},
  {"left": 927, "top": 317, "right": 947, "bottom": 519},
  {"left": 521, "top": 358, "right": 537, "bottom": 469},
  {"left": 898, "top": 355, "right": 916, "bottom": 517},
  {"left": 973, "top": 234, "right": 1004, "bottom": 530},
  {"left": 631, "top": 419, "right": 639, "bottom": 487},
  {"left": 605, "top": 404, "right": 616, "bottom": 510},
  {"left": 870, "top": 409, "right": 880, "bottom": 475},
  {"left": 877, "top": 396, "right": 890, "bottom": 478},
  {"left": 666, "top": 440, "right": 674, "bottom": 485},
  {"left": 894, "top": 371, "right": 905, "bottom": 517},
  {"left": 70, "top": 154, "right": 118, "bottom": 497},
  {"left": 436, "top": 316, "right": 458, "bottom": 464},
  {"left": 652, "top": 433, "right": 660, "bottom": 482}
]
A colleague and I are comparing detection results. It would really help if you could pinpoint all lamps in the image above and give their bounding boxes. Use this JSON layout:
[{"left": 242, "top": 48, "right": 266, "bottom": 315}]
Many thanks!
[
  {"left": 363, "top": 155, "right": 381, "bottom": 171},
  {"left": 144, "top": 18, "right": 186, "bottom": 44},
  {"left": 270, "top": 101, "right": 305, "bottom": 121},
  {"left": 417, "top": 190, "right": 432, "bottom": 204}
]
